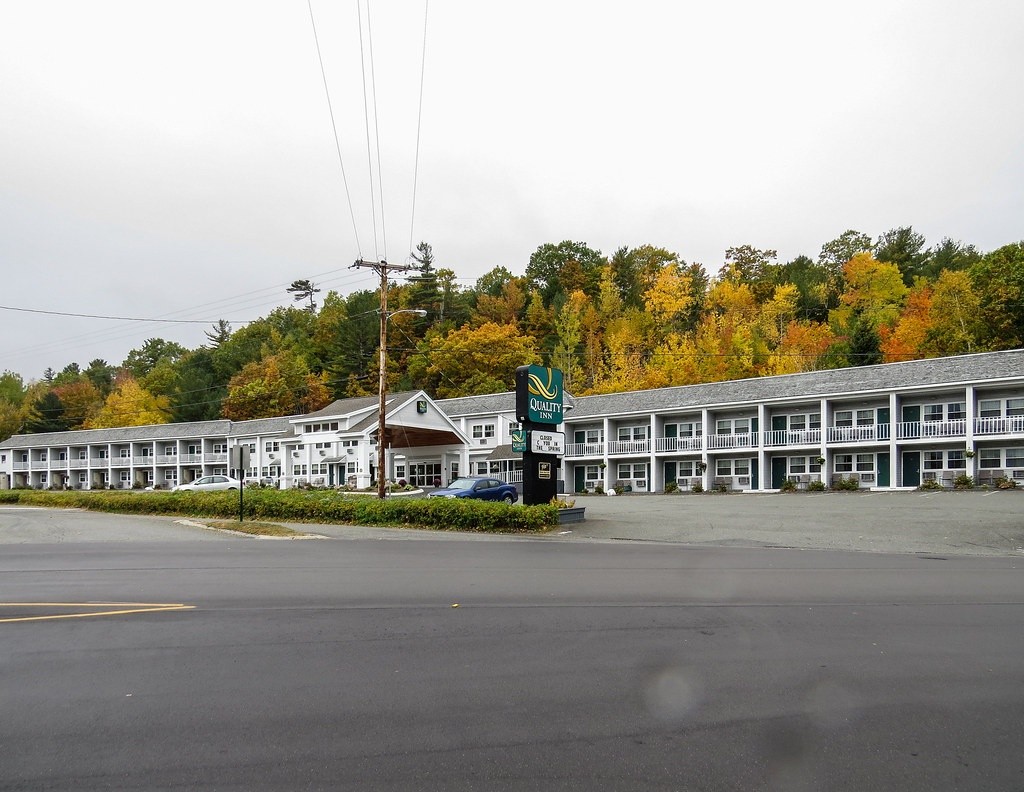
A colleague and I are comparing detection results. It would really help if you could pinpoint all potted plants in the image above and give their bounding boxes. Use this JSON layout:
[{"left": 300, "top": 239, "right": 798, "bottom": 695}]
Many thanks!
[
  {"left": 964, "top": 451, "right": 976, "bottom": 458},
  {"left": 698, "top": 463, "right": 707, "bottom": 470},
  {"left": 600, "top": 463, "right": 606, "bottom": 469},
  {"left": 817, "top": 458, "right": 825, "bottom": 463}
]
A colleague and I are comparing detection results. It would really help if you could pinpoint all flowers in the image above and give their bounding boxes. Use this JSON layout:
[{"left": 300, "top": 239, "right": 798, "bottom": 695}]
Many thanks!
[
  {"left": 399, "top": 480, "right": 407, "bottom": 484},
  {"left": 433, "top": 479, "right": 441, "bottom": 484}
]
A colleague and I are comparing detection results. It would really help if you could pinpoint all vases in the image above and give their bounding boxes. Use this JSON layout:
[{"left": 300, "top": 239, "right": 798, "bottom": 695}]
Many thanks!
[
  {"left": 435, "top": 484, "right": 439, "bottom": 488},
  {"left": 401, "top": 484, "right": 405, "bottom": 487}
]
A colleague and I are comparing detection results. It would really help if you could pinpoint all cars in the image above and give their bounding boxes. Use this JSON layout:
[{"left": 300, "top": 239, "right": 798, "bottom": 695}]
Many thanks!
[
  {"left": 170, "top": 474, "right": 241, "bottom": 491},
  {"left": 426, "top": 476, "right": 519, "bottom": 506}
]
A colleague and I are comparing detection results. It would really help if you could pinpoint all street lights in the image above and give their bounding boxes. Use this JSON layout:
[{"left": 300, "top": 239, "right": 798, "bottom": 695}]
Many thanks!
[{"left": 377, "top": 309, "right": 428, "bottom": 499}]
